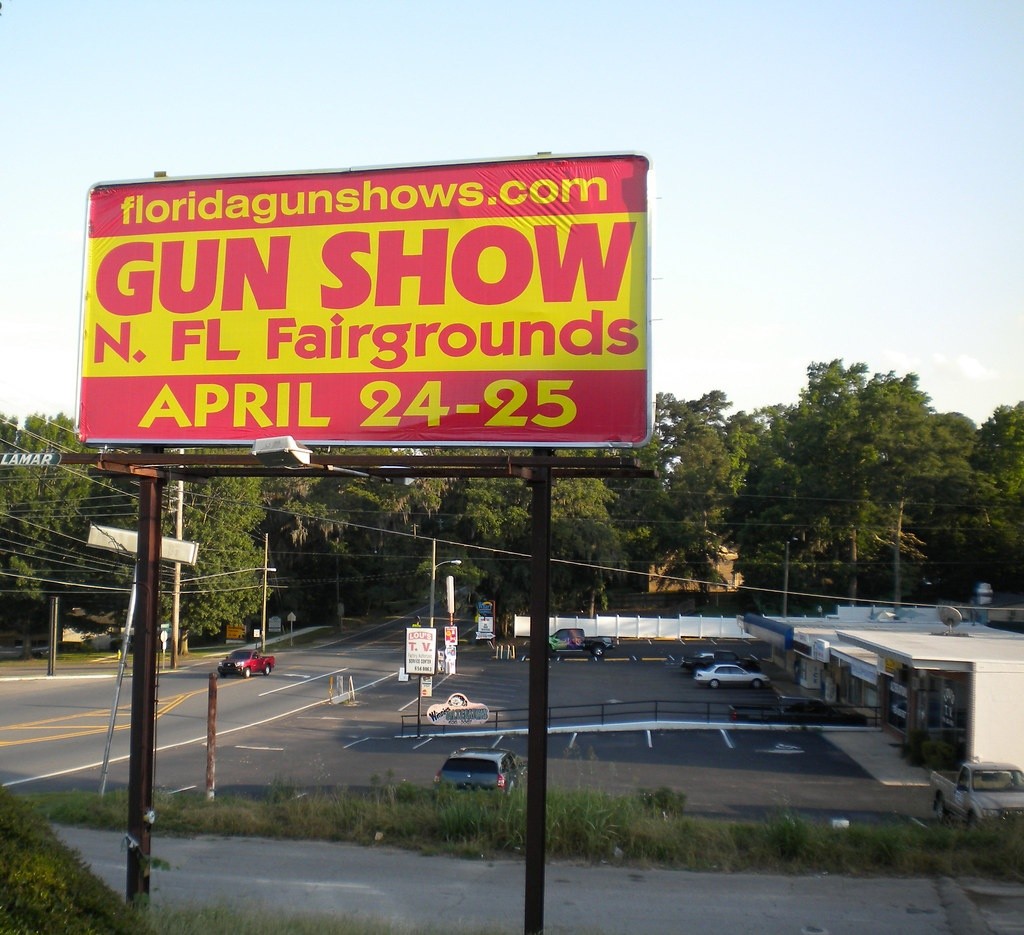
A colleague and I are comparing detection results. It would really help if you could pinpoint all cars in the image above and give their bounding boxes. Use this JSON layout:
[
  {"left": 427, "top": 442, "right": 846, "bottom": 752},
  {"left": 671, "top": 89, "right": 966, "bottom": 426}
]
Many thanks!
[
  {"left": 693, "top": 664, "right": 771, "bottom": 690},
  {"left": 433, "top": 746, "right": 527, "bottom": 799}
]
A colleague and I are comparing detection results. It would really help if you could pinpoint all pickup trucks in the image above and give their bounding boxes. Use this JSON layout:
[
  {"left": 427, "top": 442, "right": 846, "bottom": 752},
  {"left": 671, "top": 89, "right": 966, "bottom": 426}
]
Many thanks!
[
  {"left": 216, "top": 648, "right": 277, "bottom": 678},
  {"left": 728, "top": 694, "right": 868, "bottom": 727},
  {"left": 928, "top": 760, "right": 1024, "bottom": 828},
  {"left": 680, "top": 649, "right": 762, "bottom": 675},
  {"left": 524, "top": 629, "right": 614, "bottom": 657}
]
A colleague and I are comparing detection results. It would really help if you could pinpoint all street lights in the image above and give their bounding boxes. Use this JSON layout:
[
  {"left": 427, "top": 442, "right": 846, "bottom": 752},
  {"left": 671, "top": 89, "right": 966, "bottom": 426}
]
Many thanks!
[{"left": 429, "top": 560, "right": 462, "bottom": 627}]
